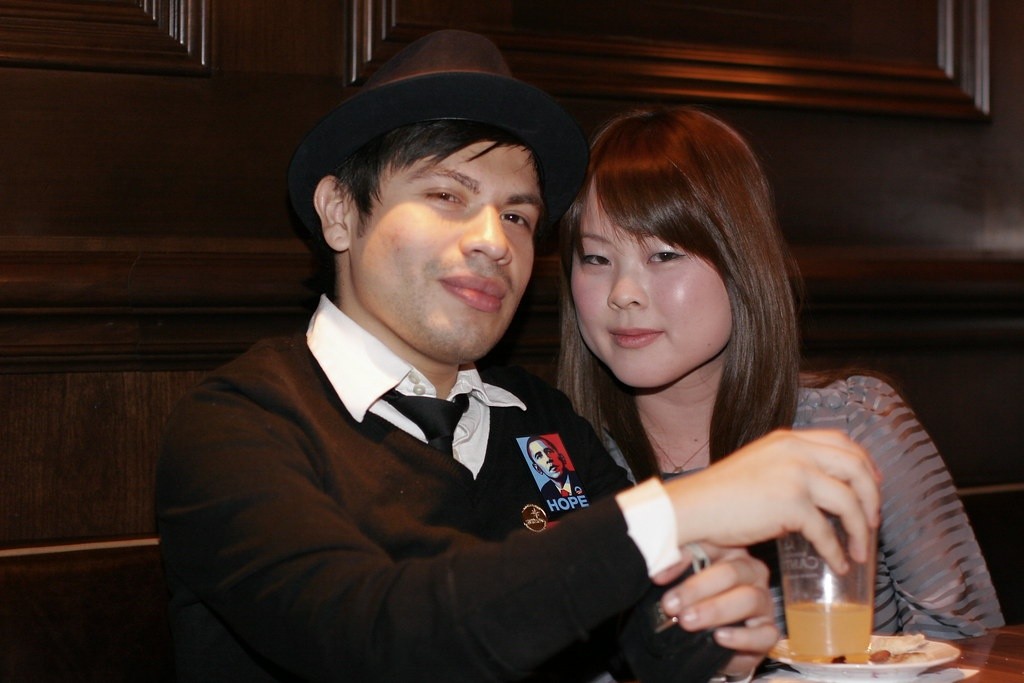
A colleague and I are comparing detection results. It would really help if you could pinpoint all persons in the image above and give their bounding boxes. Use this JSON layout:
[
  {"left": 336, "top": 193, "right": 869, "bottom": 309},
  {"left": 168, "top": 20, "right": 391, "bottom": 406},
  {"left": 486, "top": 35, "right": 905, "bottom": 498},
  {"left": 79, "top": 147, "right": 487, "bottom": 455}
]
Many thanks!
[
  {"left": 556, "top": 103, "right": 1006, "bottom": 641},
  {"left": 153, "top": 28, "right": 884, "bottom": 683}
]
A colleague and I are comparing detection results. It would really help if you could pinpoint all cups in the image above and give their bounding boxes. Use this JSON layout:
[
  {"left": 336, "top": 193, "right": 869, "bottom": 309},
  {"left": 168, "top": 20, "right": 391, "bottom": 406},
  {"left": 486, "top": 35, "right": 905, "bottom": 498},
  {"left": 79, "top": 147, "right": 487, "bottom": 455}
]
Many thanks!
[{"left": 773, "top": 479, "right": 878, "bottom": 663}]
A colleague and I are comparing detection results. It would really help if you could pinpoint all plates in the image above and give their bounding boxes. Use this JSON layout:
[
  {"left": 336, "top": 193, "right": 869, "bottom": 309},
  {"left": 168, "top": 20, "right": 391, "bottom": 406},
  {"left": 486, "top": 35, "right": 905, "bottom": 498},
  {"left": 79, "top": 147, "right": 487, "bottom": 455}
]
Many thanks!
[{"left": 765, "top": 635, "right": 961, "bottom": 681}]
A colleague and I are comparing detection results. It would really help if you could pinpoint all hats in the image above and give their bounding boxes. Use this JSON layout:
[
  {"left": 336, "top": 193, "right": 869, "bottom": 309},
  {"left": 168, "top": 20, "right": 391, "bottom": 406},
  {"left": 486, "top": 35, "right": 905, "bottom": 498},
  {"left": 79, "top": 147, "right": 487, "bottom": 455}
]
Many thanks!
[{"left": 285, "top": 31, "right": 592, "bottom": 267}]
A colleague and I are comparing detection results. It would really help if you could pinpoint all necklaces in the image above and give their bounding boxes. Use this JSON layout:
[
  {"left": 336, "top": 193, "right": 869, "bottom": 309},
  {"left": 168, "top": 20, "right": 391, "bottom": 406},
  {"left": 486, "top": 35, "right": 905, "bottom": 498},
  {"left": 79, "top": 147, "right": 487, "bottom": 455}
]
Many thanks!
[{"left": 643, "top": 425, "right": 709, "bottom": 473}]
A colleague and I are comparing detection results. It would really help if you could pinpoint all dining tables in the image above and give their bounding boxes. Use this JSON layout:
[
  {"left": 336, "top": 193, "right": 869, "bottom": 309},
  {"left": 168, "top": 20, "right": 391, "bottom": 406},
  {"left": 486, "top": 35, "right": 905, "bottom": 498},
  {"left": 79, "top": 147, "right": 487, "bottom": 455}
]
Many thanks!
[{"left": 749, "top": 624, "right": 1024, "bottom": 683}]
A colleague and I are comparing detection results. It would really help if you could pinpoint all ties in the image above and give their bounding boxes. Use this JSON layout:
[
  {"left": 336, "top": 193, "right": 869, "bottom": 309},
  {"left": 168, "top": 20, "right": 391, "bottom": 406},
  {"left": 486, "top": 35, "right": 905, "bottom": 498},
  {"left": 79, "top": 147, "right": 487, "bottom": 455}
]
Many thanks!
[{"left": 381, "top": 386, "right": 470, "bottom": 461}]
